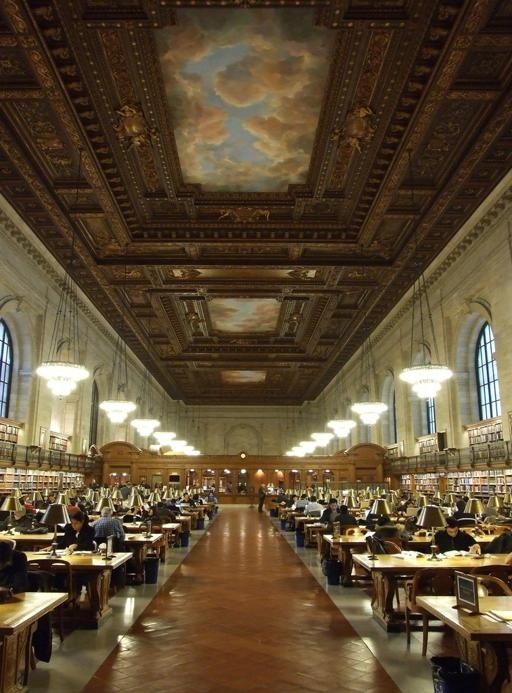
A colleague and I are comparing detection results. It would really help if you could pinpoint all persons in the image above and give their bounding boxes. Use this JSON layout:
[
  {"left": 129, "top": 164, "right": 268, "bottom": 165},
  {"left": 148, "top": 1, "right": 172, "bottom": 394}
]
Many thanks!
[
  {"left": 258, "top": 483, "right": 512, "bottom": 554},
  {"left": 1, "top": 482, "right": 217, "bottom": 663}
]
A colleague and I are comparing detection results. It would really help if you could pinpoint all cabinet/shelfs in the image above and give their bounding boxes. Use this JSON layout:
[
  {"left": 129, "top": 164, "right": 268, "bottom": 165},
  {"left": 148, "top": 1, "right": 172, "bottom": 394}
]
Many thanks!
[
  {"left": 384, "top": 410, "right": 512, "bottom": 495},
  {"left": 0, "top": 417, "right": 95, "bottom": 496}
]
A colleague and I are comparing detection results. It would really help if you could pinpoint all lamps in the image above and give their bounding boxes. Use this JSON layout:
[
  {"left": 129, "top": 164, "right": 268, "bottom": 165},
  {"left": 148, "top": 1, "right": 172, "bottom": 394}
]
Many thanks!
[{"left": 34, "top": 144, "right": 202, "bottom": 458}]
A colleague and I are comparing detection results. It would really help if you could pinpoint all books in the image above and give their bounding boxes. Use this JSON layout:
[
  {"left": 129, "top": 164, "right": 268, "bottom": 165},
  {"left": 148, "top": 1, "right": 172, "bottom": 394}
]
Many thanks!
[
  {"left": 412, "top": 422, "right": 512, "bottom": 507},
  {"left": 1, "top": 465, "right": 86, "bottom": 490}
]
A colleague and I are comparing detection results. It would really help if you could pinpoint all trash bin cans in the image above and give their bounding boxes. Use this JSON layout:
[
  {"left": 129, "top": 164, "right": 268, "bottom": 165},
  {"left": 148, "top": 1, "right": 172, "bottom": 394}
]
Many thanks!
[
  {"left": 270, "top": 509, "right": 274, "bottom": 516},
  {"left": 181, "top": 533, "right": 188, "bottom": 546},
  {"left": 197, "top": 518, "right": 204, "bottom": 529},
  {"left": 144, "top": 557, "right": 159, "bottom": 584},
  {"left": 281, "top": 519, "right": 285, "bottom": 529},
  {"left": 430, "top": 657, "right": 479, "bottom": 693},
  {"left": 296, "top": 533, "right": 304, "bottom": 547},
  {"left": 323, "top": 560, "right": 342, "bottom": 585}
]
